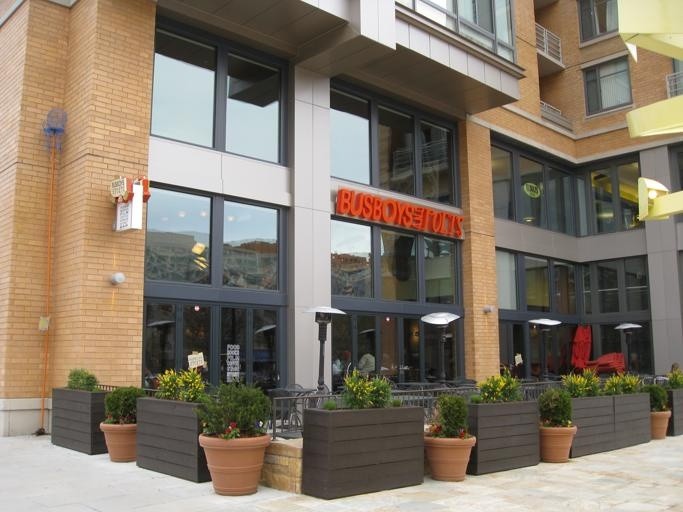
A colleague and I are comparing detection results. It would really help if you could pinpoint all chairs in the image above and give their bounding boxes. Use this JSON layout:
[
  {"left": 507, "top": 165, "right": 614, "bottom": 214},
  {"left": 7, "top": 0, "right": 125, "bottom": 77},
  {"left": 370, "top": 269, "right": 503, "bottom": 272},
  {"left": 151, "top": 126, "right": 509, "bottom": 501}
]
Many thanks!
[
  {"left": 305, "top": 375, "right": 671, "bottom": 418},
  {"left": 265, "top": 383, "right": 329, "bottom": 438}
]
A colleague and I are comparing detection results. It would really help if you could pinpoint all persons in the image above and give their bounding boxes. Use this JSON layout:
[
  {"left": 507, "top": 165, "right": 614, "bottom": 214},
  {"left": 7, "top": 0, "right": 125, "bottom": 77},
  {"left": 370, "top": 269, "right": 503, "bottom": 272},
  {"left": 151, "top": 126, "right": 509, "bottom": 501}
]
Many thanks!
[
  {"left": 331, "top": 353, "right": 344, "bottom": 376},
  {"left": 358, "top": 351, "right": 375, "bottom": 375}
]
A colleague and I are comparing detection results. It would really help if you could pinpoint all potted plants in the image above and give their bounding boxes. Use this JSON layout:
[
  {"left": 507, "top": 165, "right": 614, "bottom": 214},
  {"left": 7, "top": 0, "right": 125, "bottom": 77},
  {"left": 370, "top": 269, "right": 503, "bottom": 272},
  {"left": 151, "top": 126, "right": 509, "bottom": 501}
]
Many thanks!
[
  {"left": 427, "top": 390, "right": 478, "bottom": 483},
  {"left": 603, "top": 374, "right": 654, "bottom": 449},
  {"left": 466, "top": 364, "right": 540, "bottom": 475},
  {"left": 651, "top": 385, "right": 671, "bottom": 438},
  {"left": 51, "top": 365, "right": 272, "bottom": 495},
  {"left": 301, "top": 364, "right": 427, "bottom": 500},
  {"left": 560, "top": 363, "right": 614, "bottom": 458},
  {"left": 662, "top": 370, "right": 682, "bottom": 436},
  {"left": 538, "top": 386, "right": 579, "bottom": 466}
]
